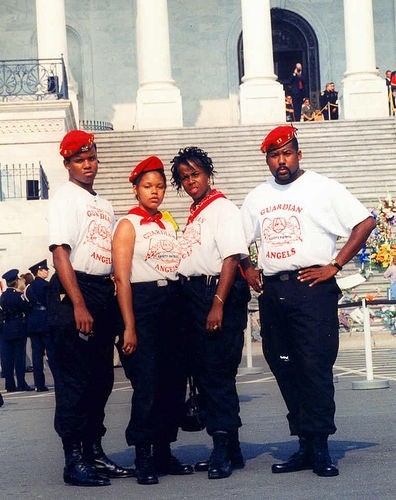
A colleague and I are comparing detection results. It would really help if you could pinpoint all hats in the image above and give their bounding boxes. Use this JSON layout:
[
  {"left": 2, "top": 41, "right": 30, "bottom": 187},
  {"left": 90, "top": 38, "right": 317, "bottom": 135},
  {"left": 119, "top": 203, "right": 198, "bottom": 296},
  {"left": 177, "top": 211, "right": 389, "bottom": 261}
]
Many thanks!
[
  {"left": 2, "top": 269, "right": 21, "bottom": 281},
  {"left": 59, "top": 130, "right": 95, "bottom": 158},
  {"left": 129, "top": 156, "right": 164, "bottom": 183},
  {"left": 29, "top": 259, "right": 49, "bottom": 276},
  {"left": 260, "top": 126, "right": 296, "bottom": 153}
]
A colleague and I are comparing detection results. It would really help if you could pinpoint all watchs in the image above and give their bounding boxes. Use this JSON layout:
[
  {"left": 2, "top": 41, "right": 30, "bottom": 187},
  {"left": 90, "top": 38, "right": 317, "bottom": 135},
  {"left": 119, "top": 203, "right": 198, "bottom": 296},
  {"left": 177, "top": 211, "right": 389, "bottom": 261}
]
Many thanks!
[{"left": 330, "top": 259, "right": 342, "bottom": 270}]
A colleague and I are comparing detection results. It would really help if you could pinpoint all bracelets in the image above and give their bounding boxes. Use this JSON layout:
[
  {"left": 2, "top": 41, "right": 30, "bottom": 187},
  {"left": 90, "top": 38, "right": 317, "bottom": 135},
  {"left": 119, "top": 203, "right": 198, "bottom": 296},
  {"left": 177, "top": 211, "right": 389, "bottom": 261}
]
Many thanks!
[{"left": 214, "top": 294, "right": 225, "bottom": 306}]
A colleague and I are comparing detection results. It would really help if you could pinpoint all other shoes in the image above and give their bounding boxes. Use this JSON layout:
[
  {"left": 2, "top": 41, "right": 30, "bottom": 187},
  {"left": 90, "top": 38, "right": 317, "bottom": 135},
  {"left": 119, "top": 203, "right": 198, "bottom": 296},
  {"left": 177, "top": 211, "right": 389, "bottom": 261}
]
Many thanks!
[
  {"left": 37, "top": 385, "right": 49, "bottom": 392},
  {"left": 16, "top": 384, "right": 35, "bottom": 391},
  {"left": 27, "top": 367, "right": 33, "bottom": 371},
  {"left": 6, "top": 386, "right": 17, "bottom": 392}
]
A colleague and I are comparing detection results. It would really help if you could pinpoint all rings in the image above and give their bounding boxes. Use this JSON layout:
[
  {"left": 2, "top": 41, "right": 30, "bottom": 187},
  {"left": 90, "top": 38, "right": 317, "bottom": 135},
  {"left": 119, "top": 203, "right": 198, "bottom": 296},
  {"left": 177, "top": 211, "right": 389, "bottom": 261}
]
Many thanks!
[
  {"left": 214, "top": 324, "right": 217, "bottom": 330},
  {"left": 128, "top": 346, "right": 131, "bottom": 349}
]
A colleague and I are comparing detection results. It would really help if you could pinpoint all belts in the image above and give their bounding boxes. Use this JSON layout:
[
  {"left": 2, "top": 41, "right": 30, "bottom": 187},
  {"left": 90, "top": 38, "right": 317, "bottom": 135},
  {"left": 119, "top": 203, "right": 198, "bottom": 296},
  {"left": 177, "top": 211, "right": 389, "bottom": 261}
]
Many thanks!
[
  {"left": 76, "top": 273, "right": 114, "bottom": 285},
  {"left": 179, "top": 274, "right": 219, "bottom": 286},
  {"left": 132, "top": 279, "right": 178, "bottom": 287},
  {"left": 260, "top": 272, "right": 298, "bottom": 282},
  {"left": 32, "top": 304, "right": 46, "bottom": 310}
]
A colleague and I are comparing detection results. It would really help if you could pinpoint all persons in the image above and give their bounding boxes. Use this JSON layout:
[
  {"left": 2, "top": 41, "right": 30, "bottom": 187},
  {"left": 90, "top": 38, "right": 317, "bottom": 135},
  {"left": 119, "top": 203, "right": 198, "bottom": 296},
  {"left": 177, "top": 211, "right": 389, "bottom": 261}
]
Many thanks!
[
  {"left": 46, "top": 130, "right": 136, "bottom": 485},
  {"left": 170, "top": 147, "right": 252, "bottom": 479},
  {"left": 240, "top": 125, "right": 376, "bottom": 478},
  {"left": 284, "top": 61, "right": 395, "bottom": 119},
  {"left": 0, "top": 258, "right": 58, "bottom": 391},
  {"left": 112, "top": 156, "right": 193, "bottom": 484}
]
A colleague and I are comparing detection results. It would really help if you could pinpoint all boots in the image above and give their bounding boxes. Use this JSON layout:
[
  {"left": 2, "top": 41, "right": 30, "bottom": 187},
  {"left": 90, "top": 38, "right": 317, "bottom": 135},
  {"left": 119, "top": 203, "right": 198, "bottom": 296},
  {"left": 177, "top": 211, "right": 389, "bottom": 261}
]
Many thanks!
[
  {"left": 63, "top": 442, "right": 111, "bottom": 485},
  {"left": 152, "top": 444, "right": 193, "bottom": 473},
  {"left": 135, "top": 444, "right": 159, "bottom": 483},
  {"left": 271, "top": 439, "right": 313, "bottom": 473},
  {"left": 83, "top": 442, "right": 135, "bottom": 477},
  {"left": 194, "top": 432, "right": 245, "bottom": 471},
  {"left": 207, "top": 431, "right": 232, "bottom": 478},
  {"left": 312, "top": 440, "right": 339, "bottom": 476}
]
